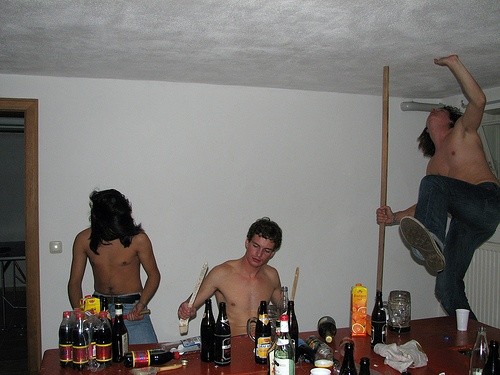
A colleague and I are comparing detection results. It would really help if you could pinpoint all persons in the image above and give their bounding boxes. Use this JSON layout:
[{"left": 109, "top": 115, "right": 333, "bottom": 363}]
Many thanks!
[
  {"left": 68, "top": 189, "right": 162, "bottom": 344},
  {"left": 375, "top": 55, "right": 500, "bottom": 321},
  {"left": 177, "top": 217, "right": 281, "bottom": 336}
]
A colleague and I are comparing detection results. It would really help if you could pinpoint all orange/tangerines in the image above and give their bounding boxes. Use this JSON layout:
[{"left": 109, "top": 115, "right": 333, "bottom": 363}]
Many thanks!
[{"left": 352, "top": 306, "right": 367, "bottom": 335}]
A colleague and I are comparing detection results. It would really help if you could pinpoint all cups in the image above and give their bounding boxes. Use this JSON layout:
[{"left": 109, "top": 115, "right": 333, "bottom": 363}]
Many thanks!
[
  {"left": 387, "top": 291, "right": 411, "bottom": 334},
  {"left": 309, "top": 359, "right": 334, "bottom": 375},
  {"left": 455, "top": 308, "right": 470, "bottom": 331}
]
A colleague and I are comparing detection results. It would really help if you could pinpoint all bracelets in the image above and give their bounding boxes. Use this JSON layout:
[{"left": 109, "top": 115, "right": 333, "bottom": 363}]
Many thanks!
[{"left": 392, "top": 212, "right": 397, "bottom": 225}]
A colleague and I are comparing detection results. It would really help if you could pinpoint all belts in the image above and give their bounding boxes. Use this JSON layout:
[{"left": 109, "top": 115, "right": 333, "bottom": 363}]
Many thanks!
[{"left": 92, "top": 294, "right": 141, "bottom": 304}]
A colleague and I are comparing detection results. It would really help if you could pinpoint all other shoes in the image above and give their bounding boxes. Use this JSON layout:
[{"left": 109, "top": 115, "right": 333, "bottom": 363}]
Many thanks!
[{"left": 400, "top": 216, "right": 446, "bottom": 274}]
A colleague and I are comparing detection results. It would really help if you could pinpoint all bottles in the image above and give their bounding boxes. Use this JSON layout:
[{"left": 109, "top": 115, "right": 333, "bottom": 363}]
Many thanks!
[
  {"left": 370, "top": 291, "right": 387, "bottom": 346},
  {"left": 124, "top": 348, "right": 180, "bottom": 367},
  {"left": 482, "top": 339, "right": 500, "bottom": 375},
  {"left": 212, "top": 302, "right": 232, "bottom": 366},
  {"left": 249, "top": 286, "right": 314, "bottom": 375},
  {"left": 200, "top": 299, "right": 216, "bottom": 362},
  {"left": 318, "top": 316, "right": 336, "bottom": 343},
  {"left": 358, "top": 357, "right": 371, "bottom": 375},
  {"left": 339, "top": 343, "right": 357, "bottom": 375},
  {"left": 58, "top": 298, "right": 130, "bottom": 369},
  {"left": 306, "top": 335, "right": 337, "bottom": 365},
  {"left": 469, "top": 325, "right": 489, "bottom": 375}
]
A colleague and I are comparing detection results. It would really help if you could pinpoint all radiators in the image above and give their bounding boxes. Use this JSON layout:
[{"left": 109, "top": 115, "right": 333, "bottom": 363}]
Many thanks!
[{"left": 462, "top": 241, "right": 500, "bottom": 330}]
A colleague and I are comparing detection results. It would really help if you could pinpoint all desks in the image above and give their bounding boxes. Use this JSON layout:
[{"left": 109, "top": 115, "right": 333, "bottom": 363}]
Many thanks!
[
  {"left": 38, "top": 315, "right": 500, "bottom": 375},
  {"left": 0, "top": 241, "right": 26, "bottom": 331}
]
